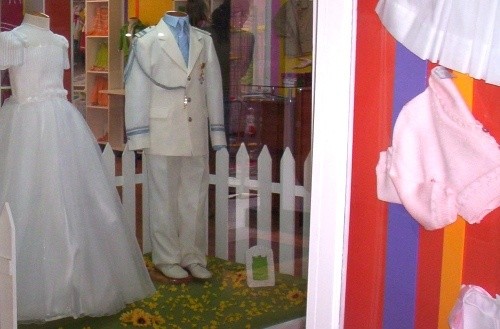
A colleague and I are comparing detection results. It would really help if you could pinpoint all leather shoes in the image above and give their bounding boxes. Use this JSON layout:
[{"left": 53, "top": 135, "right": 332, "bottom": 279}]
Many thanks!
[
  {"left": 155, "top": 264, "right": 189, "bottom": 279},
  {"left": 187, "top": 263, "right": 212, "bottom": 279}
]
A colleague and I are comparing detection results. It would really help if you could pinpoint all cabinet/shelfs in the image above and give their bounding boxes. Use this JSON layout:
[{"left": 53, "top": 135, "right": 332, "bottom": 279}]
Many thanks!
[{"left": 84, "top": 1, "right": 127, "bottom": 152}]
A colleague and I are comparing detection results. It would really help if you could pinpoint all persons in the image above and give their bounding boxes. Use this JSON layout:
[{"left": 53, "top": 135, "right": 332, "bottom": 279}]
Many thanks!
[
  {"left": 0, "top": 10, "right": 114, "bottom": 329},
  {"left": 120, "top": 9, "right": 230, "bottom": 281}
]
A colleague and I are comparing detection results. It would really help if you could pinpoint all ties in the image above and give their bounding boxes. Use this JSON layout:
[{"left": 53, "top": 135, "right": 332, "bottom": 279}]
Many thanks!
[{"left": 178, "top": 19, "right": 189, "bottom": 64}]
